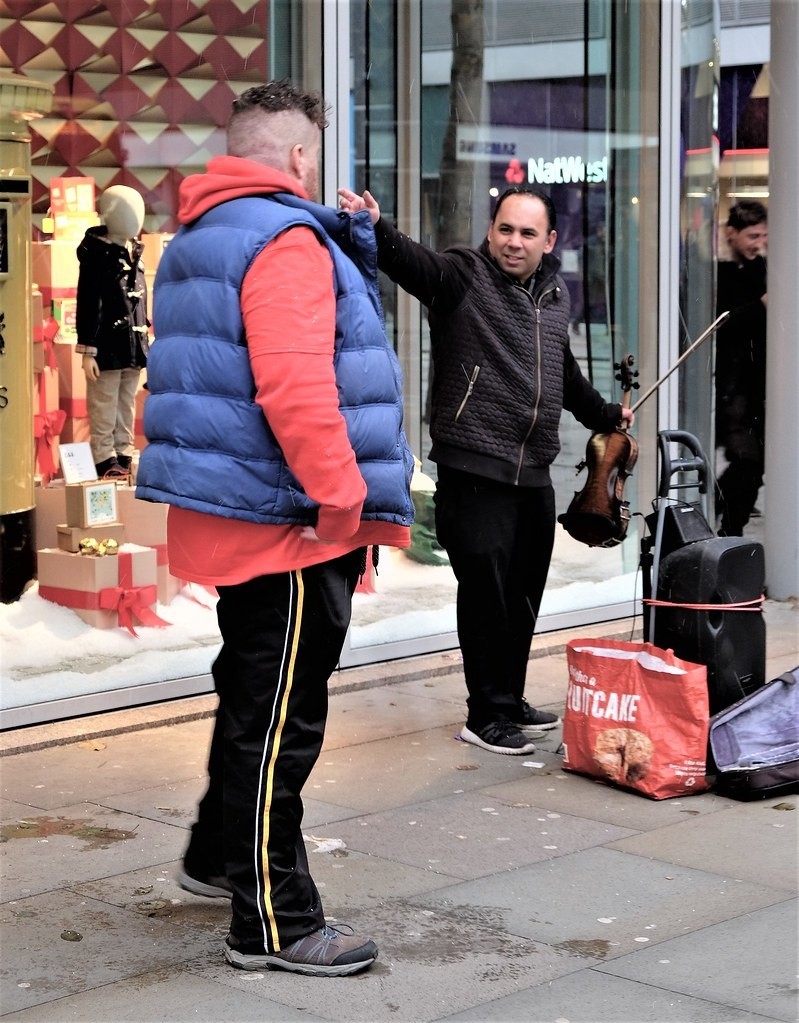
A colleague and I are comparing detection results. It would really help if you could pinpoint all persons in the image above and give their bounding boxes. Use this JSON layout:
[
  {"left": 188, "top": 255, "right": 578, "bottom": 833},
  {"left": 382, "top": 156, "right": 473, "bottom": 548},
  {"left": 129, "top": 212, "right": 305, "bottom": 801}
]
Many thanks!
[
  {"left": 133, "top": 74, "right": 415, "bottom": 975},
  {"left": 336, "top": 182, "right": 636, "bottom": 756},
  {"left": 681, "top": 199, "right": 769, "bottom": 539},
  {"left": 74, "top": 184, "right": 152, "bottom": 480}
]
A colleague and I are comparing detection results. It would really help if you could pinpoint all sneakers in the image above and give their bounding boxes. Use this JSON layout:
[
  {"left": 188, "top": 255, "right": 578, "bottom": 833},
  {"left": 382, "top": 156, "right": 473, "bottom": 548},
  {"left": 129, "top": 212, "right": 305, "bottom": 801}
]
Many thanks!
[
  {"left": 461, "top": 713, "right": 535, "bottom": 755},
  {"left": 224, "top": 918, "right": 379, "bottom": 976},
  {"left": 508, "top": 697, "right": 561, "bottom": 730},
  {"left": 181, "top": 861, "right": 236, "bottom": 900}
]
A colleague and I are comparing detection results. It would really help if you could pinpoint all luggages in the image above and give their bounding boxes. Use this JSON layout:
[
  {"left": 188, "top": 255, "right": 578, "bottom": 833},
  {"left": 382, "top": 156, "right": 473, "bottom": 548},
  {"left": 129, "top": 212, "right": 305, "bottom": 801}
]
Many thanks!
[{"left": 636, "top": 429, "right": 766, "bottom": 714}]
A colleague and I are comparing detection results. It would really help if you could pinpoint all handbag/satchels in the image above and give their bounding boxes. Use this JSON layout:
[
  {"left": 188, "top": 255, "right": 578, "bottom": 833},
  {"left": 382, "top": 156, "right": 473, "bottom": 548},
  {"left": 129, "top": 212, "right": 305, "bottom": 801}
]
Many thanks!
[
  {"left": 707, "top": 664, "right": 798, "bottom": 801},
  {"left": 566, "top": 639, "right": 709, "bottom": 801}
]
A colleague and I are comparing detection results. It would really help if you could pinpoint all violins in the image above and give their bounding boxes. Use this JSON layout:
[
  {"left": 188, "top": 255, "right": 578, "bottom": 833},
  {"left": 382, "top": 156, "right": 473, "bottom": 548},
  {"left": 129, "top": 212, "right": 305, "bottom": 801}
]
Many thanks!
[{"left": 559, "top": 355, "right": 640, "bottom": 549}]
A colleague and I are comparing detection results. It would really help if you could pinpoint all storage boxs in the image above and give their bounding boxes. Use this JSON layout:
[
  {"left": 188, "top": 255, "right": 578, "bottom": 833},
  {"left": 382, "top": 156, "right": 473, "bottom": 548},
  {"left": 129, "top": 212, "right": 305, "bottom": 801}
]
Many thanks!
[
  {"left": 66, "top": 479, "right": 118, "bottom": 528},
  {"left": 55, "top": 524, "right": 126, "bottom": 552}
]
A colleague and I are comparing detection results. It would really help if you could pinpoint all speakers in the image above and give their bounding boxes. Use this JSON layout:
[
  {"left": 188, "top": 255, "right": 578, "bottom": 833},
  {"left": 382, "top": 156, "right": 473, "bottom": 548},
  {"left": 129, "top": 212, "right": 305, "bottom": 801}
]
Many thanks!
[
  {"left": 645, "top": 501, "right": 714, "bottom": 556},
  {"left": 656, "top": 536, "right": 767, "bottom": 776}
]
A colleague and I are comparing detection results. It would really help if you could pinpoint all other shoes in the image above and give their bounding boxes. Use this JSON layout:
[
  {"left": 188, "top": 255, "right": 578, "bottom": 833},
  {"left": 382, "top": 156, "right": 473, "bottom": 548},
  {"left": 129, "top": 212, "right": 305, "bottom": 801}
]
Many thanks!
[
  {"left": 96, "top": 456, "right": 131, "bottom": 480},
  {"left": 119, "top": 456, "right": 137, "bottom": 472}
]
